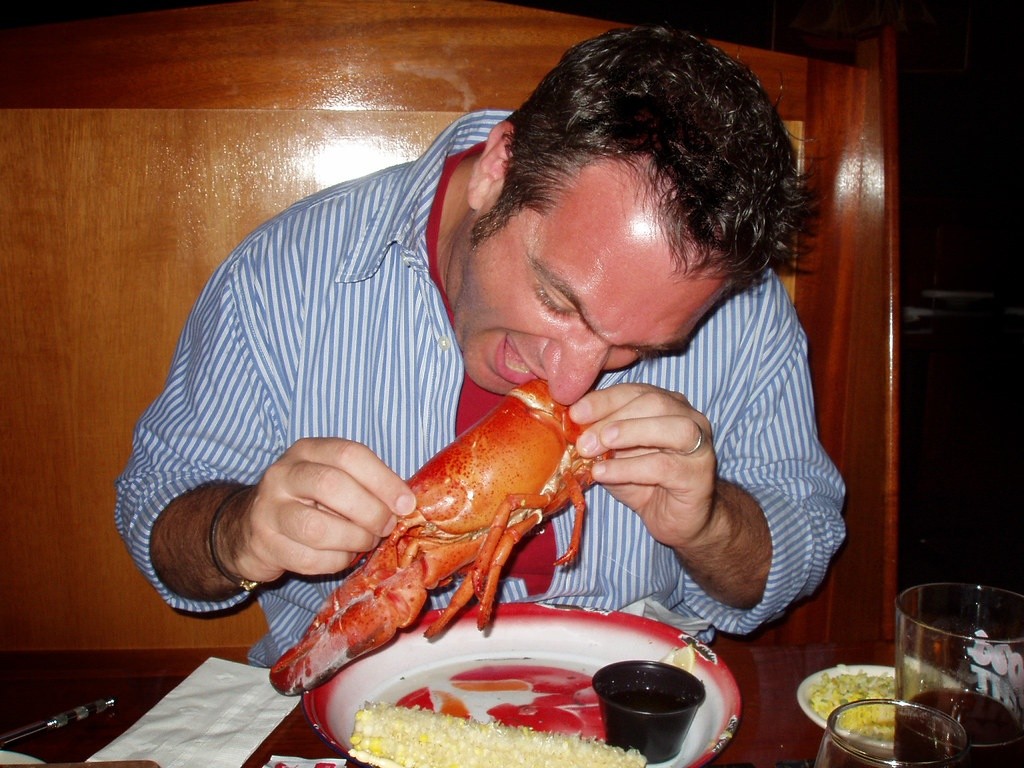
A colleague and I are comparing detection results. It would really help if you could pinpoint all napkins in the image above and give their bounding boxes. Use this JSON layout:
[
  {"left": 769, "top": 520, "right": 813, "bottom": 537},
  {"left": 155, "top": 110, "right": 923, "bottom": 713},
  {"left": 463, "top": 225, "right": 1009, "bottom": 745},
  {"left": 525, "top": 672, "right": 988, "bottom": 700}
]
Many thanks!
[{"left": 84, "top": 657, "right": 302, "bottom": 768}]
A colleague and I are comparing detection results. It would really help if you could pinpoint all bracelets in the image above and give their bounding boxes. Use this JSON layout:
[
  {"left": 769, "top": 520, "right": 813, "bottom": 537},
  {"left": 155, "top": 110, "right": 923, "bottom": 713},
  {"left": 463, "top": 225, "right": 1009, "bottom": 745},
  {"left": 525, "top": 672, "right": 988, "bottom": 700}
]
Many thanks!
[{"left": 210, "top": 486, "right": 258, "bottom": 591}]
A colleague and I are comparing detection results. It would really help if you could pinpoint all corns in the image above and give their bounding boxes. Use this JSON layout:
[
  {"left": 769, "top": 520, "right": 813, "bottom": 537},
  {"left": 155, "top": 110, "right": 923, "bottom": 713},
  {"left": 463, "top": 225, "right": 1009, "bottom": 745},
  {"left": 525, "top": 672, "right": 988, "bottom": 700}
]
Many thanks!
[{"left": 350, "top": 706, "right": 650, "bottom": 767}]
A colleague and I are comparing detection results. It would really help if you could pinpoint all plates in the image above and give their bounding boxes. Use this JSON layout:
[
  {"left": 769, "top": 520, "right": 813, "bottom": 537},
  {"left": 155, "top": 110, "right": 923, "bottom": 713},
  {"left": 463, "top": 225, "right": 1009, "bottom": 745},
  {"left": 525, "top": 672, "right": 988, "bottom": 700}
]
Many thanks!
[{"left": 797, "top": 664, "right": 897, "bottom": 759}]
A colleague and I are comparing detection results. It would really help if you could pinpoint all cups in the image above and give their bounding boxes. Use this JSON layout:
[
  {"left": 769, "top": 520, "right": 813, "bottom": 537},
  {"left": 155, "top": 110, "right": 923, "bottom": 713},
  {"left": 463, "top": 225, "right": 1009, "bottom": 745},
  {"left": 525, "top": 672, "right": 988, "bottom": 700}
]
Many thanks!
[
  {"left": 592, "top": 660, "right": 707, "bottom": 763},
  {"left": 814, "top": 699, "right": 969, "bottom": 768},
  {"left": 896, "top": 582, "right": 1022, "bottom": 766}
]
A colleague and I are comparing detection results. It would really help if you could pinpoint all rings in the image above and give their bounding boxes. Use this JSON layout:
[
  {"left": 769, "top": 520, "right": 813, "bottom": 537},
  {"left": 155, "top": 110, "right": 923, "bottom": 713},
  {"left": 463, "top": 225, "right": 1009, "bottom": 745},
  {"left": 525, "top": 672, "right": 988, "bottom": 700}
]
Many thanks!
[{"left": 682, "top": 433, "right": 702, "bottom": 454}]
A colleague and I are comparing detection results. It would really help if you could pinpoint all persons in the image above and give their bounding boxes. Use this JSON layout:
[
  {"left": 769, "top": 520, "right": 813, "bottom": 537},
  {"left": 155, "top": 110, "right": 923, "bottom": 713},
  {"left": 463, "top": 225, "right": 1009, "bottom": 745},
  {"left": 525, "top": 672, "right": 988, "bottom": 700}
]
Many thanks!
[{"left": 116, "top": 27, "right": 845, "bottom": 664}]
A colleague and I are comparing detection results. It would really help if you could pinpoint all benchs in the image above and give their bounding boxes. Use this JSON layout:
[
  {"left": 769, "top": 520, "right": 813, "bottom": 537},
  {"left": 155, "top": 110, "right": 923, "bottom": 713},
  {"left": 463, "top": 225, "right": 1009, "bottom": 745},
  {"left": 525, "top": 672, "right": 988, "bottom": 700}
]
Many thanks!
[{"left": 0, "top": 0, "right": 900, "bottom": 650}]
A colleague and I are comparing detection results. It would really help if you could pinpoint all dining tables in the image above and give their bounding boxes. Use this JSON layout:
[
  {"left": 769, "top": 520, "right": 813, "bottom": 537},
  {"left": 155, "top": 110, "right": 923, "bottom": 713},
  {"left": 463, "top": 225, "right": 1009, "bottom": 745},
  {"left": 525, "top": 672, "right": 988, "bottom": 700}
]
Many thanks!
[{"left": 0, "top": 643, "right": 945, "bottom": 768}]
